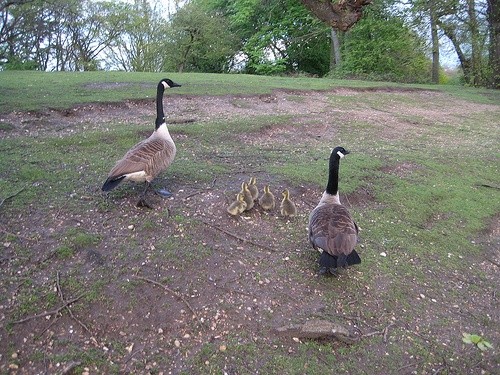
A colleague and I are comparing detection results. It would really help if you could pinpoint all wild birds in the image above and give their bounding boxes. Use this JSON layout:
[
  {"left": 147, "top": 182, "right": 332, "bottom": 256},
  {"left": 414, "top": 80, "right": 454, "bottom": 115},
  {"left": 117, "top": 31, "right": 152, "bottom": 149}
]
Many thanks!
[
  {"left": 226, "top": 193, "right": 247, "bottom": 216},
  {"left": 237, "top": 182, "right": 254, "bottom": 210},
  {"left": 280, "top": 188, "right": 297, "bottom": 220},
  {"left": 308, "top": 146, "right": 362, "bottom": 274},
  {"left": 247, "top": 176, "right": 259, "bottom": 199},
  {"left": 258, "top": 184, "right": 276, "bottom": 211},
  {"left": 102, "top": 78, "right": 183, "bottom": 209}
]
